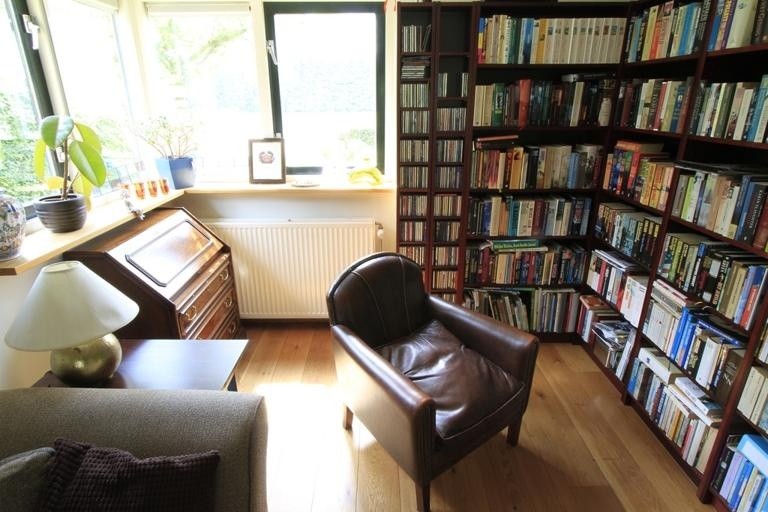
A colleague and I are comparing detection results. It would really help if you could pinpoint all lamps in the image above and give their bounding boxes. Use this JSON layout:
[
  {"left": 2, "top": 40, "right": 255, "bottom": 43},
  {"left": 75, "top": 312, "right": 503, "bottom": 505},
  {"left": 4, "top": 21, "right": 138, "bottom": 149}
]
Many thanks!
[{"left": 4, "top": 260, "right": 139, "bottom": 382}]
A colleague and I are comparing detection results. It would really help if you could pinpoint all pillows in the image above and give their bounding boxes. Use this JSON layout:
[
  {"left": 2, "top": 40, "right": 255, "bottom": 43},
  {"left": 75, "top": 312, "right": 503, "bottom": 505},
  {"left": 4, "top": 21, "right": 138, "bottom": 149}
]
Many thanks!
[
  {"left": 44, "top": 438, "right": 218, "bottom": 512},
  {"left": 0, "top": 446, "right": 54, "bottom": 511}
]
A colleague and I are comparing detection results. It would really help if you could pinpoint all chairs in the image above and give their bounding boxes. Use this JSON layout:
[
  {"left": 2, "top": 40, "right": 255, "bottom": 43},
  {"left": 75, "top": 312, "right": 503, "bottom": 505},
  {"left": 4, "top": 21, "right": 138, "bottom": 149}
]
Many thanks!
[{"left": 325, "top": 252, "right": 538, "bottom": 511}]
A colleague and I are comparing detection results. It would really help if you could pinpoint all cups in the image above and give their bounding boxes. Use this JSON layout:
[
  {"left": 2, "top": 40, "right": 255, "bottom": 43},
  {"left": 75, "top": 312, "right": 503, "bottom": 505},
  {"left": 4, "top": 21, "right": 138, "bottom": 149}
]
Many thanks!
[{"left": 120, "top": 176, "right": 169, "bottom": 200}]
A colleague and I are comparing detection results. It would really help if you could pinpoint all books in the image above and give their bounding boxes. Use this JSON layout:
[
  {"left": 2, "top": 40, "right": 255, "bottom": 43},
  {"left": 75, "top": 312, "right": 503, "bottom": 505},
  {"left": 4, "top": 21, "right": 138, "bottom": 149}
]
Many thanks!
[
  {"left": 399, "top": 24, "right": 468, "bottom": 306},
  {"left": 576, "top": 1, "right": 768, "bottom": 512},
  {"left": 469, "top": 14, "right": 632, "bottom": 333}
]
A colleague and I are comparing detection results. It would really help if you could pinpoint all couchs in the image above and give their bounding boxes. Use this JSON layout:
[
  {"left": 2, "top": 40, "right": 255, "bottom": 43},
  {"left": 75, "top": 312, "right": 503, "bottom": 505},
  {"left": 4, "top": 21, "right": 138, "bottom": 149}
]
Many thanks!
[{"left": 0, "top": 388, "right": 268, "bottom": 512}]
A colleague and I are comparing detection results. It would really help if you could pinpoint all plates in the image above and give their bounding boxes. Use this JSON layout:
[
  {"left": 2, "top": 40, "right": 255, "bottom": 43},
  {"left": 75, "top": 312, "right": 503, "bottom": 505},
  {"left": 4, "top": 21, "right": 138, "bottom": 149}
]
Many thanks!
[{"left": 291, "top": 180, "right": 320, "bottom": 187}]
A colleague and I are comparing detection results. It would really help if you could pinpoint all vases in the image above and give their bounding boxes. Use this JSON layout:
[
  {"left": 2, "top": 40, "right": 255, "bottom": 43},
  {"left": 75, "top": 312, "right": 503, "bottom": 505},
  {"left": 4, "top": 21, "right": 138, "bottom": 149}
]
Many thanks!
[{"left": 132, "top": 117, "right": 195, "bottom": 189}]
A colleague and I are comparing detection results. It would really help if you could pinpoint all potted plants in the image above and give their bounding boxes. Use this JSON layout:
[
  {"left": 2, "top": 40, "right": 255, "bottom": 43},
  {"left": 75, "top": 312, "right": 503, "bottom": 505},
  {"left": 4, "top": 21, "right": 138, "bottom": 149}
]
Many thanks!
[{"left": 32, "top": 116, "right": 106, "bottom": 232}]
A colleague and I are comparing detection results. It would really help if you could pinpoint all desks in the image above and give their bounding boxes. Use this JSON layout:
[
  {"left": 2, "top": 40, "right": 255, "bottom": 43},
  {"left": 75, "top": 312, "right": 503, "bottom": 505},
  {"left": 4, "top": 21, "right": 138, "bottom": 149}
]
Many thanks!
[{"left": 30, "top": 339, "right": 249, "bottom": 391}]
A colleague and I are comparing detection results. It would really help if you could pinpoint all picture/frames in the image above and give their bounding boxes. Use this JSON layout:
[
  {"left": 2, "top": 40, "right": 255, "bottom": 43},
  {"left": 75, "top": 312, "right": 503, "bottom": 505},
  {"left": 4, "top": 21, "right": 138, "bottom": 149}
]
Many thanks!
[{"left": 248, "top": 138, "right": 285, "bottom": 184}]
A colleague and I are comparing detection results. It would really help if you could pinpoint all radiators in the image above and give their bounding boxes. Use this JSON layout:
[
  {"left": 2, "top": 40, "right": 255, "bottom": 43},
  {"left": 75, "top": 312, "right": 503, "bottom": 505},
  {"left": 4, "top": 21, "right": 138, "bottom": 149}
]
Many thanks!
[{"left": 199, "top": 217, "right": 383, "bottom": 318}]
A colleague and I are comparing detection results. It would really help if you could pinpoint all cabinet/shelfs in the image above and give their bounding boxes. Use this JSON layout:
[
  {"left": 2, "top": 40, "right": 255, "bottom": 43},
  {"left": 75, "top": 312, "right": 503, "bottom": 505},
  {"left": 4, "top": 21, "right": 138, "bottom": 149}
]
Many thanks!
[
  {"left": 623, "top": 3, "right": 768, "bottom": 489},
  {"left": 702, "top": 280, "right": 768, "bottom": 512},
  {"left": 398, "top": 3, "right": 644, "bottom": 342},
  {"left": 62, "top": 207, "right": 241, "bottom": 339},
  {"left": 573, "top": 2, "right": 713, "bottom": 392}
]
